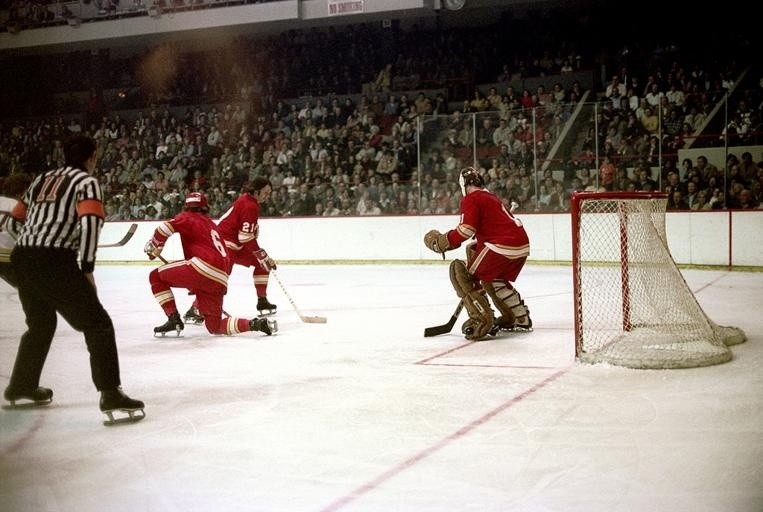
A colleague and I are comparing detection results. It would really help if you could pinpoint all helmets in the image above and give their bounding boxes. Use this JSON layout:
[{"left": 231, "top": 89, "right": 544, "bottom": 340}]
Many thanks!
[
  {"left": 183, "top": 192, "right": 209, "bottom": 209},
  {"left": 461, "top": 170, "right": 485, "bottom": 187}
]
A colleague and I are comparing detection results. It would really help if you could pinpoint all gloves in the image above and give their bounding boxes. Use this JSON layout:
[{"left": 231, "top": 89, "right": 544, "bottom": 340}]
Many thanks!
[
  {"left": 144, "top": 237, "right": 164, "bottom": 261},
  {"left": 253, "top": 247, "right": 278, "bottom": 273}
]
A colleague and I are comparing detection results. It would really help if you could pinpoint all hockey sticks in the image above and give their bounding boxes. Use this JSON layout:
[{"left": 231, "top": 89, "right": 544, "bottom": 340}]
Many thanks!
[
  {"left": 95, "top": 223, "right": 138, "bottom": 248},
  {"left": 271, "top": 268, "right": 327, "bottom": 324},
  {"left": 424, "top": 201, "right": 519, "bottom": 337}
]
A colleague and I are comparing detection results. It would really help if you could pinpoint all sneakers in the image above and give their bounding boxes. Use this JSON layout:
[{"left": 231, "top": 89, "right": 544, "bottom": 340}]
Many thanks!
[
  {"left": 514, "top": 315, "right": 533, "bottom": 329},
  {"left": 97, "top": 385, "right": 146, "bottom": 412},
  {"left": 4, "top": 382, "right": 56, "bottom": 401}
]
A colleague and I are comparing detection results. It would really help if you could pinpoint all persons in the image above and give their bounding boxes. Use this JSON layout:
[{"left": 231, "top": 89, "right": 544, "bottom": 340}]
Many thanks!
[
  {"left": 4, "top": 132, "right": 146, "bottom": 412},
  {"left": 2, "top": 0, "right": 762, "bottom": 220},
  {"left": 144, "top": 190, "right": 273, "bottom": 335},
  {"left": 184, "top": 178, "right": 278, "bottom": 322},
  {"left": 2, "top": 170, "right": 31, "bottom": 291},
  {"left": 423, "top": 165, "right": 533, "bottom": 340}
]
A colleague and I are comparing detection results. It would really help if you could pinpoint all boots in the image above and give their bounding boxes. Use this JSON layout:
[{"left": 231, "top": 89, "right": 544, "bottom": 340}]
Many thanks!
[
  {"left": 185, "top": 305, "right": 206, "bottom": 321},
  {"left": 252, "top": 317, "right": 272, "bottom": 336},
  {"left": 256, "top": 296, "right": 278, "bottom": 311},
  {"left": 154, "top": 312, "right": 185, "bottom": 332}
]
